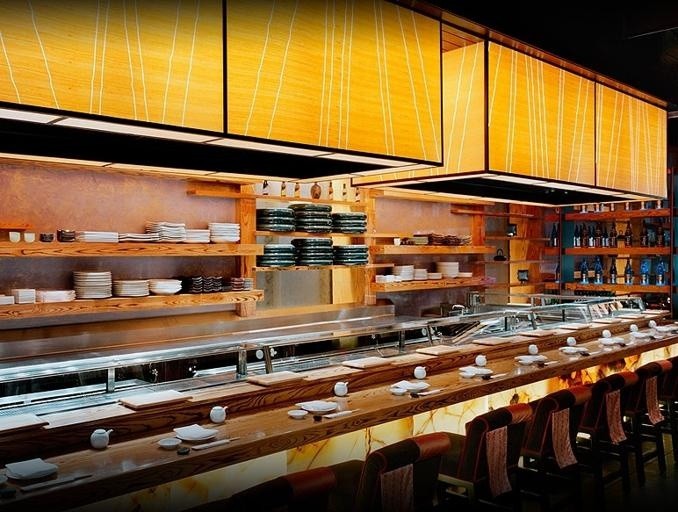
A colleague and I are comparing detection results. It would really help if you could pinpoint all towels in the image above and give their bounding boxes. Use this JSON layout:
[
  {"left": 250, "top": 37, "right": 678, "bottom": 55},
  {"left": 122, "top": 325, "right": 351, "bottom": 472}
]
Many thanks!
[
  {"left": 171, "top": 424, "right": 219, "bottom": 439},
  {"left": 5, "top": 458, "right": 59, "bottom": 481},
  {"left": 556, "top": 324, "right": 678, "bottom": 356}
]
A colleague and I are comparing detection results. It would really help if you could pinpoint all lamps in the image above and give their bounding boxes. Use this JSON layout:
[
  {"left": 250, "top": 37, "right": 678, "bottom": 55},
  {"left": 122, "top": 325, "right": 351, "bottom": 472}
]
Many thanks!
[
  {"left": 538, "top": 84, "right": 667, "bottom": 208},
  {"left": 226, "top": 0, "right": 444, "bottom": 179},
  {"left": 0, "top": 1, "right": 225, "bottom": 161},
  {"left": 350, "top": 38, "right": 598, "bottom": 197}
]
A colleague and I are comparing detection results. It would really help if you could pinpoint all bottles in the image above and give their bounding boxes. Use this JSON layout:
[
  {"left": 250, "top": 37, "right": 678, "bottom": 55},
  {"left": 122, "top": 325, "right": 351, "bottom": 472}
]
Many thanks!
[
  {"left": 602, "top": 320, "right": 657, "bottom": 338},
  {"left": 414, "top": 366, "right": 427, "bottom": 379},
  {"left": 474, "top": 354, "right": 488, "bottom": 367},
  {"left": 262, "top": 179, "right": 361, "bottom": 201},
  {"left": 528, "top": 344, "right": 538, "bottom": 354},
  {"left": 90, "top": 428, "right": 109, "bottom": 450},
  {"left": 334, "top": 382, "right": 348, "bottom": 396},
  {"left": 210, "top": 406, "right": 226, "bottom": 425},
  {"left": 566, "top": 336, "right": 577, "bottom": 347},
  {"left": 549, "top": 219, "right": 666, "bottom": 288},
  {"left": 580, "top": 200, "right": 664, "bottom": 214}
]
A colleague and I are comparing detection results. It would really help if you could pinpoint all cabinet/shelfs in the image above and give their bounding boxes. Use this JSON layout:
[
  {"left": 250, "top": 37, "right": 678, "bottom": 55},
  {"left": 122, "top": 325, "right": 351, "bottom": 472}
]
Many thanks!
[
  {"left": 478, "top": 209, "right": 558, "bottom": 286},
  {"left": 255, "top": 193, "right": 394, "bottom": 272},
  {"left": 562, "top": 210, "right": 672, "bottom": 294},
  {"left": 367, "top": 242, "right": 498, "bottom": 294},
  {"left": 0, "top": 232, "right": 267, "bottom": 317}
]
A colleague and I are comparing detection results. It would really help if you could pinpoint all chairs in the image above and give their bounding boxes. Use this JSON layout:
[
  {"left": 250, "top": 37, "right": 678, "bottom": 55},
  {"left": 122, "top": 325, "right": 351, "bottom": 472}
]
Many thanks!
[
  {"left": 642, "top": 356, "right": 677, "bottom": 464},
  {"left": 465, "top": 385, "right": 592, "bottom": 512},
  {"left": 327, "top": 431, "right": 451, "bottom": 512},
  {"left": 438, "top": 403, "right": 533, "bottom": 512},
  {"left": 190, "top": 466, "right": 337, "bottom": 512},
  {"left": 623, "top": 359, "right": 672, "bottom": 485},
  {"left": 574, "top": 371, "right": 640, "bottom": 503}
]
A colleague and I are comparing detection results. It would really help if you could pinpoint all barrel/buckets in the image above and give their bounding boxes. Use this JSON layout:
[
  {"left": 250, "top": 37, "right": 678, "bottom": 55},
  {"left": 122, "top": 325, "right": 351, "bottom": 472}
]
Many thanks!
[
  {"left": 579, "top": 258, "right": 589, "bottom": 284},
  {"left": 593, "top": 258, "right": 604, "bottom": 285},
  {"left": 654, "top": 260, "right": 666, "bottom": 286},
  {"left": 639, "top": 261, "right": 650, "bottom": 286}
]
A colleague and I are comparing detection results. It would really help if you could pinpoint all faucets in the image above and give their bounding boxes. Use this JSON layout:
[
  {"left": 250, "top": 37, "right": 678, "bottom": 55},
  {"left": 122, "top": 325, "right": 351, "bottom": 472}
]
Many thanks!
[{"left": 452, "top": 303, "right": 465, "bottom": 315}]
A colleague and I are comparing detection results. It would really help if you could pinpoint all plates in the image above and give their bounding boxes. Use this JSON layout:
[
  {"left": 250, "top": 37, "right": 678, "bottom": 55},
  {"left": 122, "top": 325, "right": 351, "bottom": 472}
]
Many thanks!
[
  {"left": 174, "top": 273, "right": 221, "bottom": 294},
  {"left": 34, "top": 288, "right": 77, "bottom": 302},
  {"left": 458, "top": 372, "right": 475, "bottom": 378},
  {"left": 599, "top": 339, "right": 624, "bottom": 346},
  {"left": 517, "top": 357, "right": 547, "bottom": 365},
  {"left": 230, "top": 276, "right": 254, "bottom": 289},
  {"left": 400, "top": 382, "right": 430, "bottom": 393},
  {"left": 150, "top": 277, "right": 183, "bottom": 295},
  {"left": 475, "top": 372, "right": 494, "bottom": 377},
  {"left": 177, "top": 432, "right": 217, "bottom": 443},
  {"left": 10, "top": 287, "right": 36, "bottom": 305},
  {"left": 72, "top": 268, "right": 112, "bottom": 300},
  {"left": 301, "top": 401, "right": 337, "bottom": 416},
  {"left": 561, "top": 349, "right": 586, "bottom": 354},
  {"left": 0, "top": 295, "right": 14, "bottom": 306},
  {"left": 288, "top": 409, "right": 307, "bottom": 421},
  {"left": 390, "top": 388, "right": 406, "bottom": 397},
  {"left": 112, "top": 281, "right": 151, "bottom": 299},
  {"left": 6, "top": 464, "right": 59, "bottom": 480},
  {"left": 260, "top": 200, "right": 476, "bottom": 284},
  {"left": 657, "top": 326, "right": 672, "bottom": 333},
  {"left": 158, "top": 437, "right": 182, "bottom": 451},
  {"left": 55, "top": 222, "right": 241, "bottom": 244}
]
